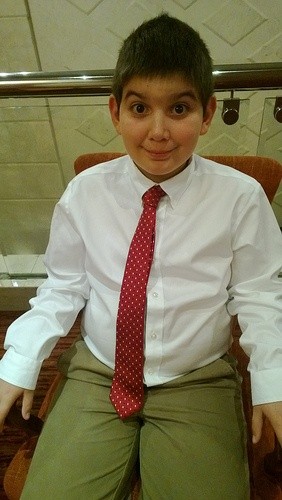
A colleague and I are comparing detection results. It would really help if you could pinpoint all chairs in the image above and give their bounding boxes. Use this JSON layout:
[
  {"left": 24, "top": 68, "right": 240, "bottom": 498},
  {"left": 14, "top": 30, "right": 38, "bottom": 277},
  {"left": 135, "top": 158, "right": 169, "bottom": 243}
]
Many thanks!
[{"left": 3, "top": 152, "right": 282, "bottom": 500}]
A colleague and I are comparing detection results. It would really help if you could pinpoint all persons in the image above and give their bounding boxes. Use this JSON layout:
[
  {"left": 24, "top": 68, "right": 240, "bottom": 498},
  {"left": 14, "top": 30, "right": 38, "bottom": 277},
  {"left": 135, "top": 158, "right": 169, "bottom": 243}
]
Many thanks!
[{"left": 0, "top": 13, "right": 282, "bottom": 500}]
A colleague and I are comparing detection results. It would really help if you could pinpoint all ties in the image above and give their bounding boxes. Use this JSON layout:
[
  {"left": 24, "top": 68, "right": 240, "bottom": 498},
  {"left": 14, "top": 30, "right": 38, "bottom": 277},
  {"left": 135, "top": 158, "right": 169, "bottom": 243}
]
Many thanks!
[{"left": 109, "top": 185, "right": 168, "bottom": 422}]
